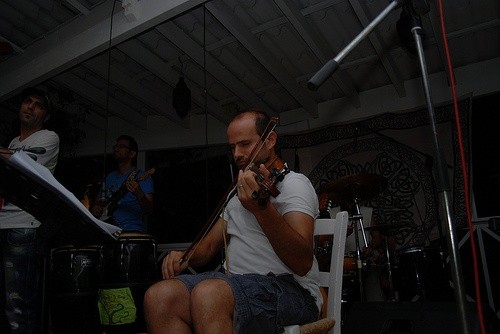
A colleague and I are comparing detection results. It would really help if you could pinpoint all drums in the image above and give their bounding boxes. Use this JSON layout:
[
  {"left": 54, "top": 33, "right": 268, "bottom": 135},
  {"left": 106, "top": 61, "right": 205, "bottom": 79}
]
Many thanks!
[
  {"left": 314, "top": 234, "right": 333, "bottom": 266},
  {"left": 111, "top": 230, "right": 160, "bottom": 283},
  {"left": 341, "top": 250, "right": 367, "bottom": 275},
  {"left": 48, "top": 241, "right": 116, "bottom": 305}
]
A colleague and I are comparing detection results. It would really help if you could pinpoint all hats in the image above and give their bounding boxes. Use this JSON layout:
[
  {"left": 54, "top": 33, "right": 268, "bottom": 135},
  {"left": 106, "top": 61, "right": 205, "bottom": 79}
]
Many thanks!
[{"left": 20, "top": 86, "right": 49, "bottom": 111}]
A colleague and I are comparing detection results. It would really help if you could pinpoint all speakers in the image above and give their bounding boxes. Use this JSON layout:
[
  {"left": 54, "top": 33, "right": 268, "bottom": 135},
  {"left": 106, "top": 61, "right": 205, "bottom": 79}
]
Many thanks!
[{"left": 396, "top": 247, "right": 445, "bottom": 301}]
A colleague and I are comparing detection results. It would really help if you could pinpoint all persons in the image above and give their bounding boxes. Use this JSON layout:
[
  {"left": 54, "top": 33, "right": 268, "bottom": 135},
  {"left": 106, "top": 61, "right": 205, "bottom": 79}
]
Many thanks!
[
  {"left": 0, "top": 88, "right": 59, "bottom": 244},
  {"left": 90, "top": 135, "right": 156, "bottom": 239},
  {"left": 144, "top": 111, "right": 324, "bottom": 334}
]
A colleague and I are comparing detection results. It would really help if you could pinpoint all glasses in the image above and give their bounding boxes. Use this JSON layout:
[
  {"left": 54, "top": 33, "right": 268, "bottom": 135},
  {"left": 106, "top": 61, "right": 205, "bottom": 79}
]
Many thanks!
[{"left": 113, "top": 144, "right": 131, "bottom": 151}]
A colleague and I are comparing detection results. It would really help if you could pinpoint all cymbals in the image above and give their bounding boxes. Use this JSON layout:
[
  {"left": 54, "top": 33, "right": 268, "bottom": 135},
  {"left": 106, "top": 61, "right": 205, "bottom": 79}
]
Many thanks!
[{"left": 358, "top": 219, "right": 412, "bottom": 232}]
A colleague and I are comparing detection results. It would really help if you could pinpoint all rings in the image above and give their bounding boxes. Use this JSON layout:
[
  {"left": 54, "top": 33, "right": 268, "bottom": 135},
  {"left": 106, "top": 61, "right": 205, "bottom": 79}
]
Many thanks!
[{"left": 238, "top": 185, "right": 244, "bottom": 188}]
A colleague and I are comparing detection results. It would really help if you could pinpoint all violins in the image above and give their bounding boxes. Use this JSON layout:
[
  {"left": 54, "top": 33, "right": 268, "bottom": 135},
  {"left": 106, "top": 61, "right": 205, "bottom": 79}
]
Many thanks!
[{"left": 250, "top": 155, "right": 286, "bottom": 202}]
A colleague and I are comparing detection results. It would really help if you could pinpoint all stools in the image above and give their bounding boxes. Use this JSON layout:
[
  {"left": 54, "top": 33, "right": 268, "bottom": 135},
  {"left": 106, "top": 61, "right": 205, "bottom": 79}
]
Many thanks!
[
  {"left": 45, "top": 245, "right": 104, "bottom": 296},
  {"left": 103, "top": 232, "right": 158, "bottom": 281}
]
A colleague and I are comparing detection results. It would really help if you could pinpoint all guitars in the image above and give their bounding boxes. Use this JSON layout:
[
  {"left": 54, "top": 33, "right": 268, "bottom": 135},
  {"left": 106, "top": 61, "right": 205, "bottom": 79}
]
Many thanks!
[{"left": 90, "top": 168, "right": 157, "bottom": 219}]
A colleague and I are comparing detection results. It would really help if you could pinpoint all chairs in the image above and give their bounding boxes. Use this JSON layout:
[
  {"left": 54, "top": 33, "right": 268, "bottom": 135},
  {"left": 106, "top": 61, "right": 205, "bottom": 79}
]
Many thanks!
[{"left": 276, "top": 212, "right": 348, "bottom": 334}]
